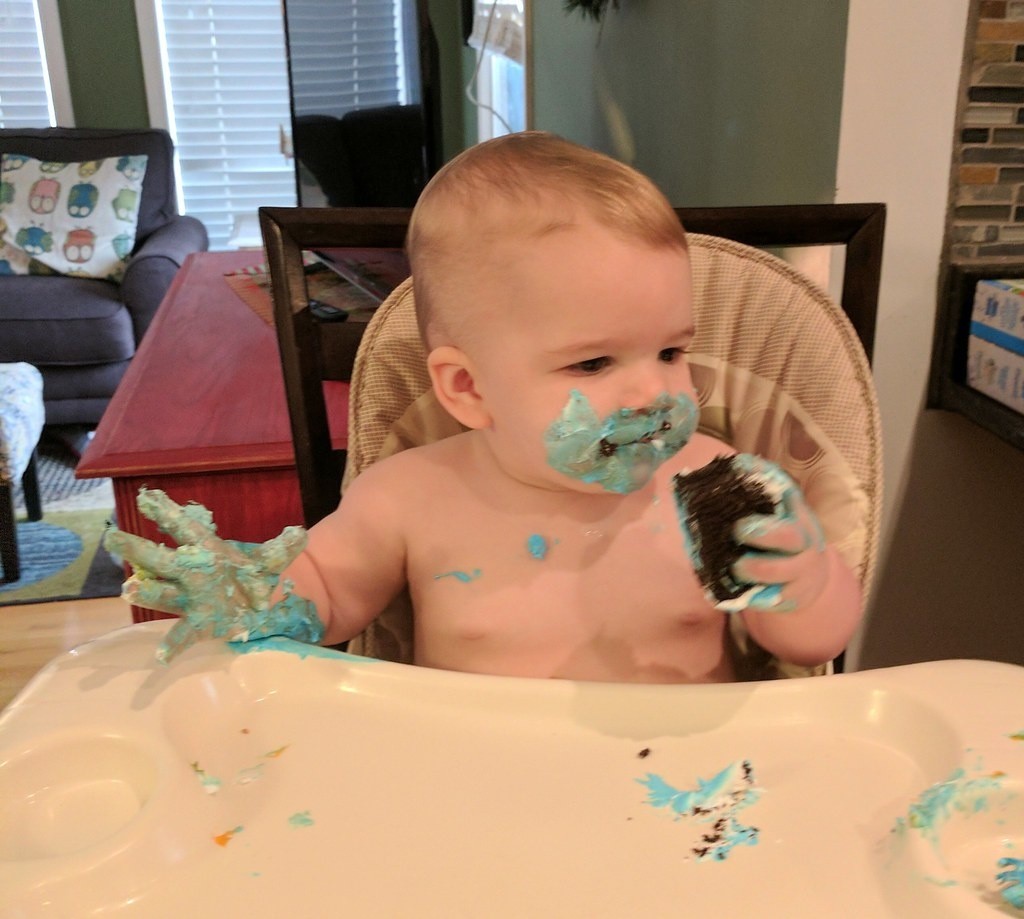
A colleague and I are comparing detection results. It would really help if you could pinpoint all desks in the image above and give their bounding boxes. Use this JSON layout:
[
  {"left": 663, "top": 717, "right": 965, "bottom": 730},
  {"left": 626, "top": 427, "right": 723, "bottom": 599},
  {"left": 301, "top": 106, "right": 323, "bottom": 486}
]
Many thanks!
[{"left": 75, "top": 250, "right": 411, "bottom": 625}]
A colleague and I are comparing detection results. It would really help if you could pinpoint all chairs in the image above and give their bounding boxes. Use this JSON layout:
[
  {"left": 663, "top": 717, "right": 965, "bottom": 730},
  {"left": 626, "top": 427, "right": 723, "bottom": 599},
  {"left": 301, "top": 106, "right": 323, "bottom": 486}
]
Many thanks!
[{"left": 0, "top": 204, "right": 1024, "bottom": 919}]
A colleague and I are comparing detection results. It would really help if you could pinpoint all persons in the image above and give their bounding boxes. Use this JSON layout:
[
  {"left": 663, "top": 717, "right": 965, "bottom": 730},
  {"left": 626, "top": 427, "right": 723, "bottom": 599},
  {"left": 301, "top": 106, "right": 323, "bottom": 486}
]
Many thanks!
[{"left": 100, "top": 131, "right": 859, "bottom": 685}]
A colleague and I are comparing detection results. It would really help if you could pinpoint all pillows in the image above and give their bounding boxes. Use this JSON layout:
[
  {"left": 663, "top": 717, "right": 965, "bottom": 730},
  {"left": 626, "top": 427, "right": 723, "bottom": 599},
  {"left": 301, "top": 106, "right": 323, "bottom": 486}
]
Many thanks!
[{"left": 0, "top": 154, "right": 147, "bottom": 284}]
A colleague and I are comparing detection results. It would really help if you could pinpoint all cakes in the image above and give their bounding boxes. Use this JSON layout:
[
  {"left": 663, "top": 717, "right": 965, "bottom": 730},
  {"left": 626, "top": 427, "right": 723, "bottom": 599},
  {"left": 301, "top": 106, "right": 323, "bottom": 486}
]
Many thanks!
[{"left": 674, "top": 456, "right": 785, "bottom": 614}]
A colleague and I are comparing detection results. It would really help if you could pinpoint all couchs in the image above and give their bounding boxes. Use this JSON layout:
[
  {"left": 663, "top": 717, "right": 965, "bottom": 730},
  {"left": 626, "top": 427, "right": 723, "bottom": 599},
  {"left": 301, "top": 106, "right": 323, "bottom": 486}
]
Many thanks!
[{"left": 0, "top": 127, "right": 209, "bottom": 427}]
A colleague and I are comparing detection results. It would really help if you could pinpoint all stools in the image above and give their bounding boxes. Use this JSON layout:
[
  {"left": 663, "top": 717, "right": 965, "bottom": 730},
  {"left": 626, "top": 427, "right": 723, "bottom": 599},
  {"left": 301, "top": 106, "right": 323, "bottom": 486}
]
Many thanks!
[{"left": 0, "top": 363, "right": 46, "bottom": 582}]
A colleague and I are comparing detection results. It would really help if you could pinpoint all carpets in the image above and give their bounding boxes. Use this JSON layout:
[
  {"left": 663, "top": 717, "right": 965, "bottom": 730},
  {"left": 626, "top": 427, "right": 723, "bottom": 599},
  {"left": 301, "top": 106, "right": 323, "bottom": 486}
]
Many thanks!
[{"left": 0, "top": 429, "right": 125, "bottom": 608}]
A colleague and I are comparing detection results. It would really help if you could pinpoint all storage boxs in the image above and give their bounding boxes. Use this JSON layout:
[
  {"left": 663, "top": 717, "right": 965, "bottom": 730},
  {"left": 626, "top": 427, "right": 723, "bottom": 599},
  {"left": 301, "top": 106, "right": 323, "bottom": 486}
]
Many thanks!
[{"left": 967, "top": 278, "right": 1023, "bottom": 415}]
